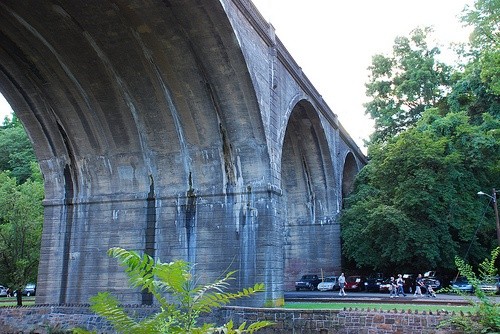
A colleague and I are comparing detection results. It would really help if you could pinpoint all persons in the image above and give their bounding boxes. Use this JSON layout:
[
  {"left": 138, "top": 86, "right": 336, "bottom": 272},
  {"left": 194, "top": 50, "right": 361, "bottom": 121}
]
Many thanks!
[
  {"left": 397, "top": 275, "right": 407, "bottom": 296},
  {"left": 338, "top": 272, "right": 347, "bottom": 296},
  {"left": 389, "top": 277, "right": 400, "bottom": 298},
  {"left": 428, "top": 285, "right": 434, "bottom": 293},
  {"left": 6, "top": 288, "right": 17, "bottom": 297},
  {"left": 413, "top": 274, "right": 425, "bottom": 298}
]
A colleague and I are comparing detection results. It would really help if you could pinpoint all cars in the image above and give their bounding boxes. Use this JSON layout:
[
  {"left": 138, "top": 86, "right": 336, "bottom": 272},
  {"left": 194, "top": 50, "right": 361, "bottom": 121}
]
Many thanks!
[
  {"left": 316, "top": 276, "right": 339, "bottom": 291},
  {"left": 421, "top": 276, "right": 443, "bottom": 292},
  {"left": 478, "top": 275, "right": 500, "bottom": 295},
  {"left": 0, "top": 285, "right": 8, "bottom": 296},
  {"left": 344, "top": 275, "right": 368, "bottom": 292},
  {"left": 450, "top": 275, "right": 475, "bottom": 293},
  {"left": 363, "top": 273, "right": 416, "bottom": 294}
]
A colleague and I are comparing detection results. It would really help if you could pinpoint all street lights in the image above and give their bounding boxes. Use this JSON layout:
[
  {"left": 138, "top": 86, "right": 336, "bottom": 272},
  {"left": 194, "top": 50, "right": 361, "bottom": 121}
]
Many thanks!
[{"left": 477, "top": 188, "right": 500, "bottom": 247}]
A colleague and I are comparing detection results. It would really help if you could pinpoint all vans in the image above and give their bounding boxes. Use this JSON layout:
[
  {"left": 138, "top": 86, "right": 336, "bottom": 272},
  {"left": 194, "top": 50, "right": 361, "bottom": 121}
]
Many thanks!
[{"left": 12, "top": 283, "right": 35, "bottom": 297}]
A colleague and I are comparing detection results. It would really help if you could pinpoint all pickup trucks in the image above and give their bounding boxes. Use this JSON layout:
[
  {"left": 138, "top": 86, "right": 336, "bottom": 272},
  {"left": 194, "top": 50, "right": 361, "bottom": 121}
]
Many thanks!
[{"left": 295, "top": 273, "right": 324, "bottom": 291}]
